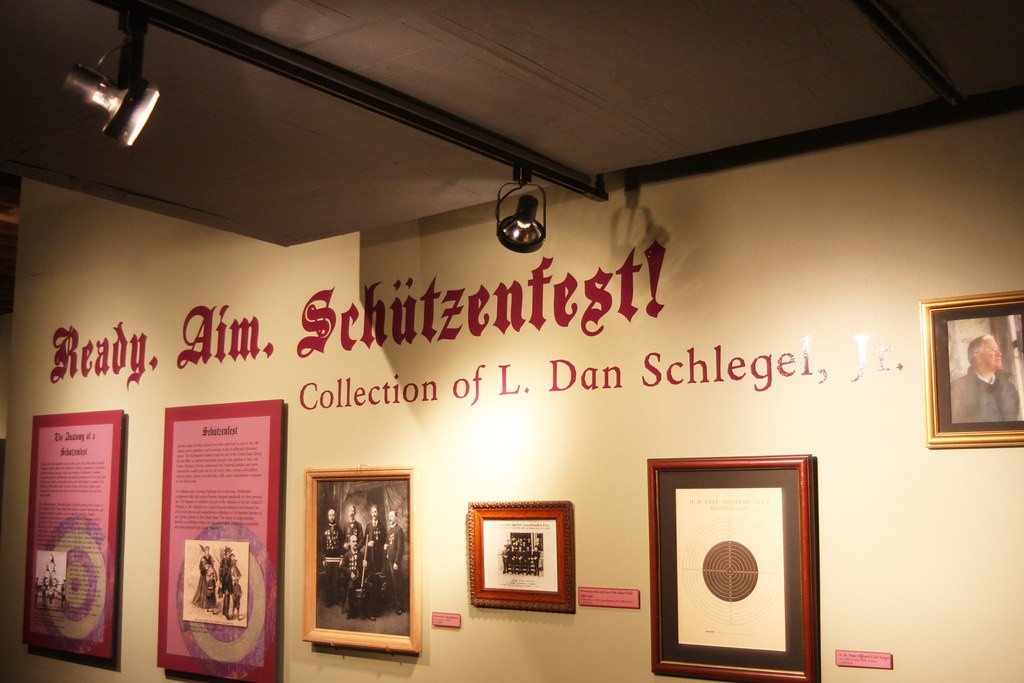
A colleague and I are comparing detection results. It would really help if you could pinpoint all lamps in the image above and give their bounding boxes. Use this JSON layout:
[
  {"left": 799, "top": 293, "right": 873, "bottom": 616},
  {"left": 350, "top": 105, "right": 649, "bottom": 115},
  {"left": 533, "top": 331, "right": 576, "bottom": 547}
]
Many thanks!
[
  {"left": 495, "top": 163, "right": 548, "bottom": 254},
  {"left": 63, "top": 8, "right": 161, "bottom": 146}
]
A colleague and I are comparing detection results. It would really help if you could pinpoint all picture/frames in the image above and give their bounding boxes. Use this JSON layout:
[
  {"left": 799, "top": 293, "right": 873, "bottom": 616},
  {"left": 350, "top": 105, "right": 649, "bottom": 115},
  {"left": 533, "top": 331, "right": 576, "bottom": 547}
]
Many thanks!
[
  {"left": 647, "top": 454, "right": 821, "bottom": 683},
  {"left": 468, "top": 501, "right": 576, "bottom": 613},
  {"left": 302, "top": 465, "right": 422, "bottom": 654},
  {"left": 918, "top": 290, "right": 1024, "bottom": 449}
]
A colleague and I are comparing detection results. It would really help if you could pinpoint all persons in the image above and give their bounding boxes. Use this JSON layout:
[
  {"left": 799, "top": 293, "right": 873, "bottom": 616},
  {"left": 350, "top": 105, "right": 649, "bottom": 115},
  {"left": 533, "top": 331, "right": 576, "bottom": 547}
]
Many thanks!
[
  {"left": 316, "top": 504, "right": 407, "bottom": 621},
  {"left": 951, "top": 335, "right": 1021, "bottom": 422},
  {"left": 192, "top": 546, "right": 243, "bottom": 622},
  {"left": 501, "top": 537, "right": 542, "bottom": 577}
]
